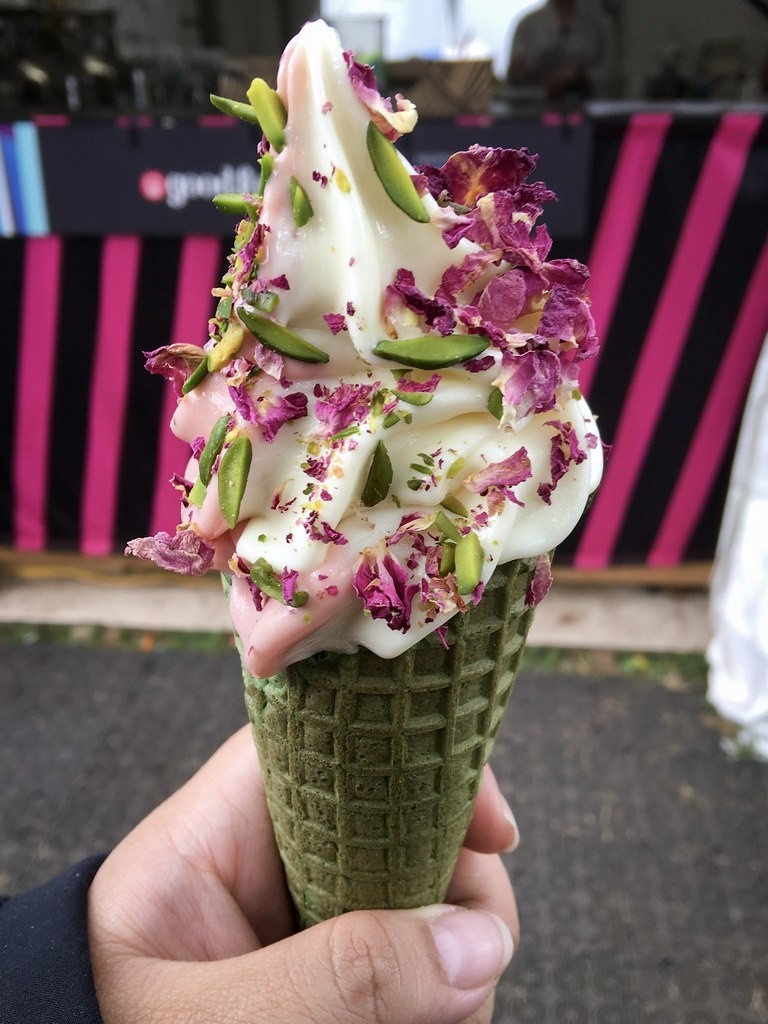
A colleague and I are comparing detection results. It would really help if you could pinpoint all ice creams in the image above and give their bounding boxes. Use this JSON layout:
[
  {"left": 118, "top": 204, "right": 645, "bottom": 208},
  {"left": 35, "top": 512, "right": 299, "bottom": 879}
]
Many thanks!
[{"left": 129, "top": 18, "right": 603, "bottom": 927}]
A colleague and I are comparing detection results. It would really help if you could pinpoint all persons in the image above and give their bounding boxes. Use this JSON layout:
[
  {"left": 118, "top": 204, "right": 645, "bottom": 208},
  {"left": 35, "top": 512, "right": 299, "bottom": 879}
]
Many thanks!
[
  {"left": 506, "top": 0, "right": 622, "bottom": 103},
  {"left": 2, "top": 715, "right": 522, "bottom": 1024}
]
